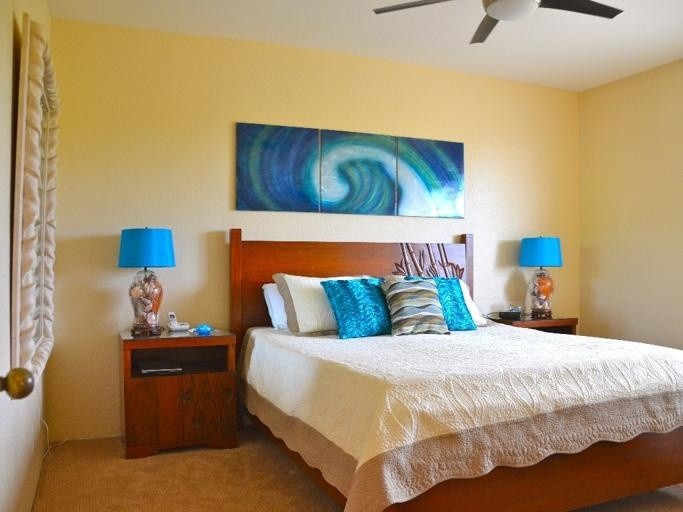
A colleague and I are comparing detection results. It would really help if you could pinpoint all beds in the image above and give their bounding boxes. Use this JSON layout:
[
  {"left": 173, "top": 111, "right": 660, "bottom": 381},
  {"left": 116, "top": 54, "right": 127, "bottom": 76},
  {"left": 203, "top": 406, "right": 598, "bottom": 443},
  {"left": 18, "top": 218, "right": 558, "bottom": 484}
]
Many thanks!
[{"left": 230, "top": 227, "right": 683, "bottom": 512}]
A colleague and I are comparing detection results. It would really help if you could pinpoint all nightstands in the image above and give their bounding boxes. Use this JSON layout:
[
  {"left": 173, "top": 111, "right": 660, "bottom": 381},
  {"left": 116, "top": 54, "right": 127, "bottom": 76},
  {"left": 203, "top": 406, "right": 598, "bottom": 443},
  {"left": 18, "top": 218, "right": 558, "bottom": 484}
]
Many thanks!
[
  {"left": 121, "top": 330, "right": 237, "bottom": 459},
  {"left": 493, "top": 318, "right": 578, "bottom": 335}
]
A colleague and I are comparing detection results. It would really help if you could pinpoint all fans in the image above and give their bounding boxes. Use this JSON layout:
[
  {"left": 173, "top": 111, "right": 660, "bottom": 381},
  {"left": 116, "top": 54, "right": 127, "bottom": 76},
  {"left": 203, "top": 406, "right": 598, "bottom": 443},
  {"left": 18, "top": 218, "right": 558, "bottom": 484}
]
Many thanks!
[{"left": 373, "top": 0, "right": 625, "bottom": 43}]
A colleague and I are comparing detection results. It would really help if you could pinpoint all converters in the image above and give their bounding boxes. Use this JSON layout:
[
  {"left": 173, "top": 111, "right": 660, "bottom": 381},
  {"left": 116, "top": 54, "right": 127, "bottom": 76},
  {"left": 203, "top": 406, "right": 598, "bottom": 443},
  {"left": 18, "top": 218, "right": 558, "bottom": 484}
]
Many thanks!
[{"left": 499, "top": 311, "right": 522, "bottom": 319}]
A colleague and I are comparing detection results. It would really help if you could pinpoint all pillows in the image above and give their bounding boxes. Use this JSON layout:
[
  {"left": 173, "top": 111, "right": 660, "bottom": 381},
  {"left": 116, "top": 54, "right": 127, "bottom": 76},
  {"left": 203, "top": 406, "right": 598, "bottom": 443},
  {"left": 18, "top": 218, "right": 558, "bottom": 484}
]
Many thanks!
[
  {"left": 272, "top": 272, "right": 487, "bottom": 336},
  {"left": 262, "top": 284, "right": 288, "bottom": 331},
  {"left": 320, "top": 277, "right": 477, "bottom": 339},
  {"left": 382, "top": 280, "right": 448, "bottom": 336}
]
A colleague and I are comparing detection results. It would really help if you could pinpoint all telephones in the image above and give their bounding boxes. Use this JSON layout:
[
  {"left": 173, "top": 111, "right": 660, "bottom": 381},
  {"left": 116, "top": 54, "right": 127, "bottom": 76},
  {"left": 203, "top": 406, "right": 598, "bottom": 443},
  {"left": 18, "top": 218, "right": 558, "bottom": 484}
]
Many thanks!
[{"left": 168, "top": 311, "right": 189, "bottom": 331}]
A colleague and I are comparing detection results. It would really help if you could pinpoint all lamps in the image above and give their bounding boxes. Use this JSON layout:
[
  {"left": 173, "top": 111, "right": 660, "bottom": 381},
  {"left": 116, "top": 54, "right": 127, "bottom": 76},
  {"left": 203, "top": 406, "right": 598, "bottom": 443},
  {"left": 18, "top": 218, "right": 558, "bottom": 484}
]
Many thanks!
[
  {"left": 482, "top": 0, "right": 541, "bottom": 21},
  {"left": 119, "top": 227, "right": 175, "bottom": 335},
  {"left": 519, "top": 236, "right": 563, "bottom": 319}
]
